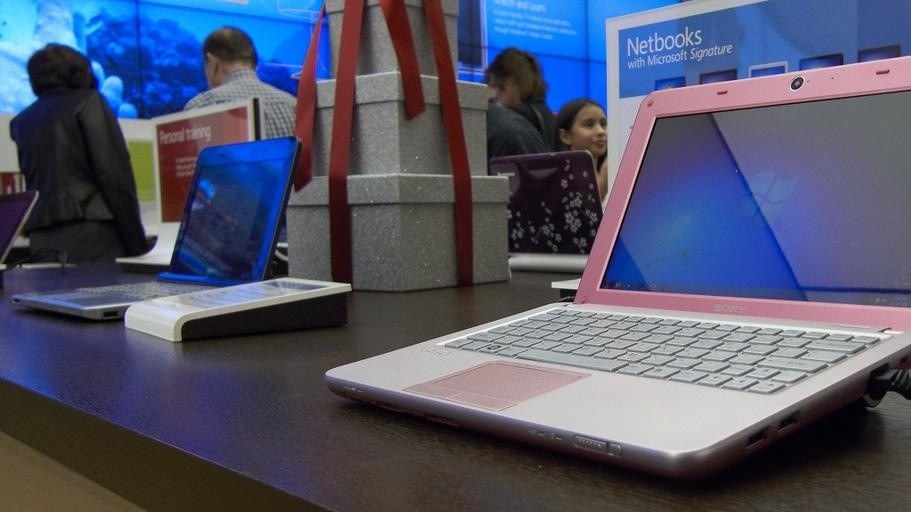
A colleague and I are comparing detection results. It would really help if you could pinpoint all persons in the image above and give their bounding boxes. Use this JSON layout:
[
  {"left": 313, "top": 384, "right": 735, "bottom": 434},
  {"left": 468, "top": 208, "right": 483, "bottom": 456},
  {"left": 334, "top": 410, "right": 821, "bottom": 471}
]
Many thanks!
[
  {"left": 484, "top": 48, "right": 556, "bottom": 153},
  {"left": 184, "top": 25, "right": 298, "bottom": 139},
  {"left": 550, "top": 97, "right": 608, "bottom": 203},
  {"left": 9, "top": 42, "right": 157, "bottom": 263},
  {"left": 487, "top": 102, "right": 546, "bottom": 160}
]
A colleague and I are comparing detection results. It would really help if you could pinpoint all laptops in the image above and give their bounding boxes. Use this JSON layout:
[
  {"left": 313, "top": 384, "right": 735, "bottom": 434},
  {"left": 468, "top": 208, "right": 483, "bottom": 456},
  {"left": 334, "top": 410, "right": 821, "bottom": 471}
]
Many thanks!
[
  {"left": 11, "top": 135, "right": 300, "bottom": 320},
  {"left": 0, "top": 190, "right": 39, "bottom": 270},
  {"left": 325, "top": 55, "right": 911, "bottom": 474},
  {"left": 654, "top": 45, "right": 901, "bottom": 91},
  {"left": 488, "top": 150, "right": 603, "bottom": 255}
]
